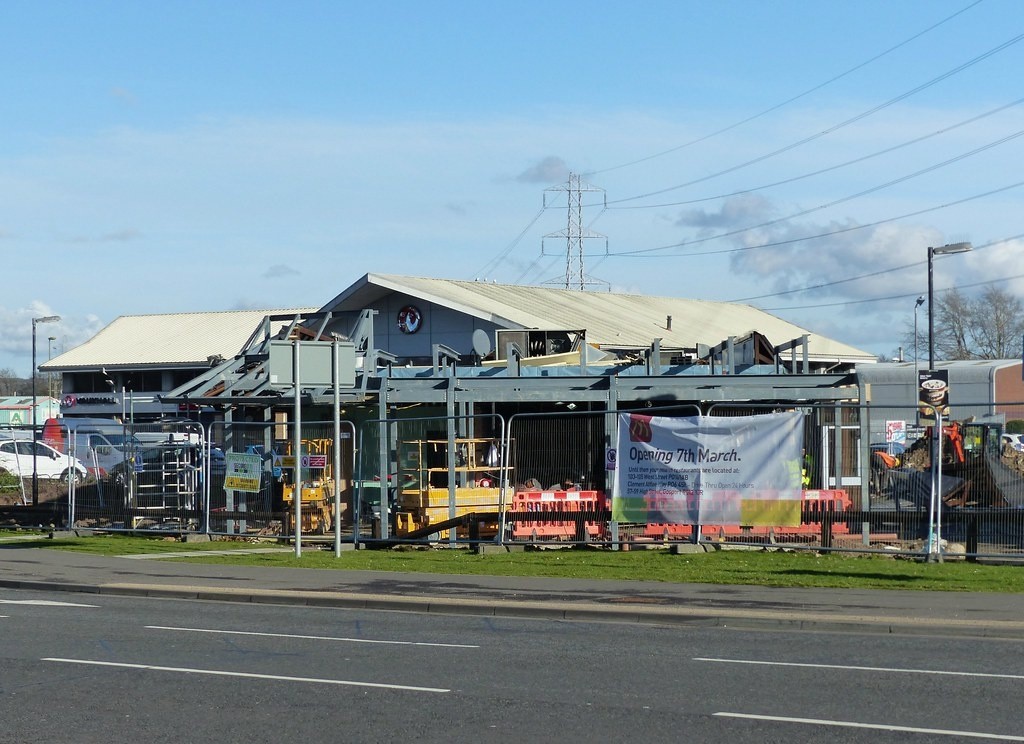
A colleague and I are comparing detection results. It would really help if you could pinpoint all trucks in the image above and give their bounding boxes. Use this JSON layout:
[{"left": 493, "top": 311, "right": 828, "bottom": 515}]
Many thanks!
[{"left": 0, "top": 418, "right": 225, "bottom": 485}]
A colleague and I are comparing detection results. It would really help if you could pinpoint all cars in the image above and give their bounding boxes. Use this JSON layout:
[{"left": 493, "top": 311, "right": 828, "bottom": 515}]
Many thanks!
[
  {"left": 870, "top": 442, "right": 906, "bottom": 456},
  {"left": 0, "top": 440, "right": 87, "bottom": 484},
  {"left": 1002, "top": 433, "right": 1024, "bottom": 452}
]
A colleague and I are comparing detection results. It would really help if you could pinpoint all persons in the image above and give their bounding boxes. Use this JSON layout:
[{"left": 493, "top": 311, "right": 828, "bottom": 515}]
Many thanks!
[
  {"left": 550, "top": 470, "right": 581, "bottom": 490},
  {"left": 785, "top": 447, "right": 812, "bottom": 490},
  {"left": 524, "top": 473, "right": 541, "bottom": 488},
  {"left": 871, "top": 451, "right": 899, "bottom": 495}
]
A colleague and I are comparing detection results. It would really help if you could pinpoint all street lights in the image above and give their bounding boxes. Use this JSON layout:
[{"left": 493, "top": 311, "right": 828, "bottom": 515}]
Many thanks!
[
  {"left": 914, "top": 296, "right": 924, "bottom": 426},
  {"left": 928, "top": 241, "right": 973, "bottom": 369},
  {"left": 32, "top": 315, "right": 61, "bottom": 505},
  {"left": 48, "top": 337, "right": 56, "bottom": 418}
]
